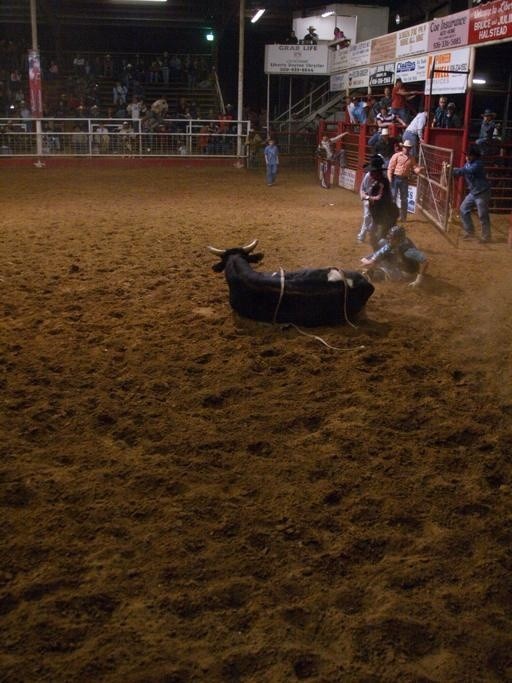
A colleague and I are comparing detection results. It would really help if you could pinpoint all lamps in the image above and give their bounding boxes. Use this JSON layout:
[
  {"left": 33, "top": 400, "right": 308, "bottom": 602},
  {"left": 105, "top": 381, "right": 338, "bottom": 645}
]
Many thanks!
[
  {"left": 206, "top": 28, "right": 216, "bottom": 42},
  {"left": 249, "top": 7, "right": 266, "bottom": 23}
]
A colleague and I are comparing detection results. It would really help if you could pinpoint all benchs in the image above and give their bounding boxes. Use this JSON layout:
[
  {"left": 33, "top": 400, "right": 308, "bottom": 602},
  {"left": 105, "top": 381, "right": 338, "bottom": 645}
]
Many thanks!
[{"left": 0, "top": 50, "right": 225, "bottom": 118}]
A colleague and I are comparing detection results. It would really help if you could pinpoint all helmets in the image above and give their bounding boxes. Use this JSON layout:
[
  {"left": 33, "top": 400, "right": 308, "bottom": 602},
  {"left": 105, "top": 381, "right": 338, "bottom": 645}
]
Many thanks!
[{"left": 386, "top": 225, "right": 405, "bottom": 247}]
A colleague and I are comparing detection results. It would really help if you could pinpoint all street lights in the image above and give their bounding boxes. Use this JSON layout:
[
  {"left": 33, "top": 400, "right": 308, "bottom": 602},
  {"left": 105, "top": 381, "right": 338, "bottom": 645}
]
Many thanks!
[
  {"left": 237, "top": 0, "right": 265, "bottom": 155},
  {"left": 322, "top": 9, "right": 337, "bottom": 26}
]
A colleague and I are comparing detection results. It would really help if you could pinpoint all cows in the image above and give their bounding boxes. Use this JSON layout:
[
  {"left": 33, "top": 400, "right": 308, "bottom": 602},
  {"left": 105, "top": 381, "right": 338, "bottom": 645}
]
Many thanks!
[{"left": 206, "top": 239, "right": 375, "bottom": 329}]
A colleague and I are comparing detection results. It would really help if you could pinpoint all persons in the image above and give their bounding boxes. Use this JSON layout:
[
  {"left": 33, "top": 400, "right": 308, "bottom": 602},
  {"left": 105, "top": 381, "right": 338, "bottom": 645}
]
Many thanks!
[
  {"left": 314, "top": 131, "right": 349, "bottom": 188},
  {"left": 343, "top": 78, "right": 504, "bottom": 289},
  {"left": 0, "top": 52, "right": 232, "bottom": 154},
  {"left": 281, "top": 31, "right": 298, "bottom": 44},
  {"left": 334, "top": 27, "right": 349, "bottom": 49},
  {"left": 303, "top": 26, "right": 319, "bottom": 44},
  {"left": 264, "top": 138, "right": 280, "bottom": 186}
]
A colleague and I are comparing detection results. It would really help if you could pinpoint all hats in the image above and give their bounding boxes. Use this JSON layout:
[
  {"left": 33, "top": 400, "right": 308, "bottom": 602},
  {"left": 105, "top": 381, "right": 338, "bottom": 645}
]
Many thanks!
[
  {"left": 307, "top": 26, "right": 316, "bottom": 30},
  {"left": 363, "top": 155, "right": 387, "bottom": 171},
  {"left": 399, "top": 140, "right": 414, "bottom": 148},
  {"left": 481, "top": 109, "right": 496, "bottom": 118},
  {"left": 462, "top": 144, "right": 479, "bottom": 157}
]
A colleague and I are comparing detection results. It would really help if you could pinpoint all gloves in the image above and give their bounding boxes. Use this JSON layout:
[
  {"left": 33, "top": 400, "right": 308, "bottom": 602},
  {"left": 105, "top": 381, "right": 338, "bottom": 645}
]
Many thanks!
[{"left": 407, "top": 273, "right": 425, "bottom": 289}]
